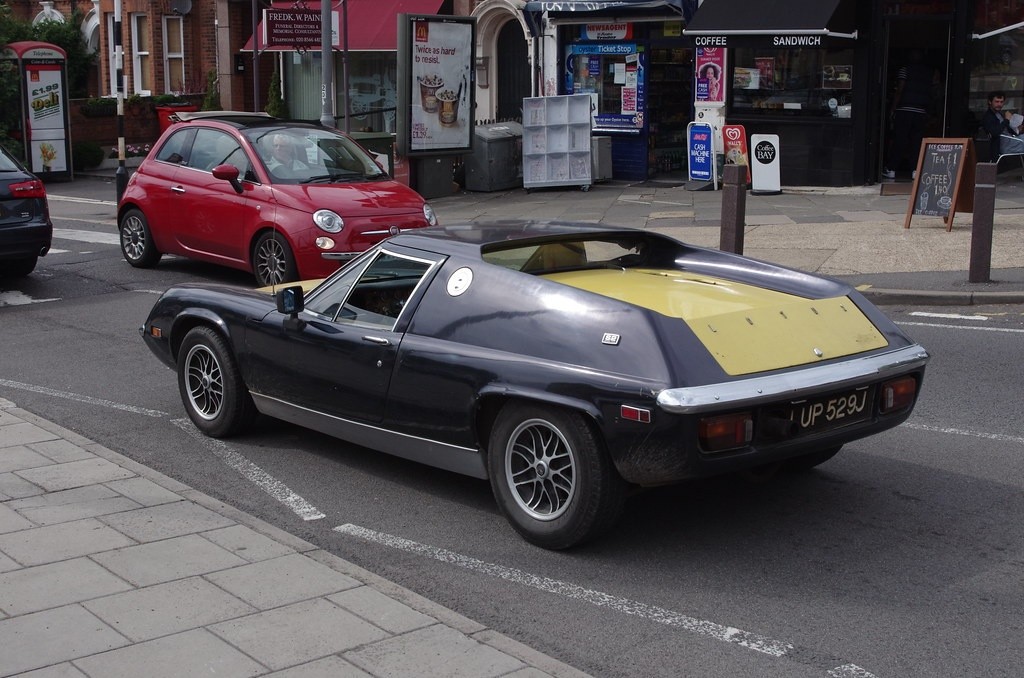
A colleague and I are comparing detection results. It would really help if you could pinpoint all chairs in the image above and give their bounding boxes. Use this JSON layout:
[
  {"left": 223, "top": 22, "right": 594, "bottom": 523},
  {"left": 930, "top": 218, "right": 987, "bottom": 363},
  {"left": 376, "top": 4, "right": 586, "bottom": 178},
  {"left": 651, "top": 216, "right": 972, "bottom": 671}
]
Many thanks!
[{"left": 988, "top": 129, "right": 1024, "bottom": 182}]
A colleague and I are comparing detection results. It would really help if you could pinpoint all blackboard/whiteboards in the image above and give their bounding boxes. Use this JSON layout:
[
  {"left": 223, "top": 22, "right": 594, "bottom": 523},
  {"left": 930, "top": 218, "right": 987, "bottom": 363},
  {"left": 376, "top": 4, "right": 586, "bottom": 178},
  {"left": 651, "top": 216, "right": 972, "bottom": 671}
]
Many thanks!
[{"left": 911, "top": 137, "right": 977, "bottom": 218}]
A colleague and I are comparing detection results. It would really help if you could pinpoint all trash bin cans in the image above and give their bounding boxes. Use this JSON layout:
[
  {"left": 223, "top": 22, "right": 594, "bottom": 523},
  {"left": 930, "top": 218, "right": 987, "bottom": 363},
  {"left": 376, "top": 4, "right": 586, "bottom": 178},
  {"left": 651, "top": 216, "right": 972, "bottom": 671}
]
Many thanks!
[
  {"left": 343, "top": 131, "right": 397, "bottom": 182},
  {"left": 155, "top": 102, "right": 199, "bottom": 136},
  {"left": 464, "top": 120, "right": 524, "bottom": 192}
]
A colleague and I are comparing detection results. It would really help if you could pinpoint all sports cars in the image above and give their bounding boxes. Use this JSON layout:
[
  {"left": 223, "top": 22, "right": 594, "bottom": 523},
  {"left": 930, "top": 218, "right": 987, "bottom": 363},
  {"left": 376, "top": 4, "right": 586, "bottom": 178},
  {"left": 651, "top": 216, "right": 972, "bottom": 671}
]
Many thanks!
[{"left": 139, "top": 220, "right": 932, "bottom": 551}]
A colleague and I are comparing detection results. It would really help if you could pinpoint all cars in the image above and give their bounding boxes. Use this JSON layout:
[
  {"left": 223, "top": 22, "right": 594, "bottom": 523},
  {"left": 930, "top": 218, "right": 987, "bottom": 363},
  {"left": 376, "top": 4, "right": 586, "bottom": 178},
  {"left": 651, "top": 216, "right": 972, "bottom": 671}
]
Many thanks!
[
  {"left": 115, "top": 110, "right": 438, "bottom": 287},
  {"left": 1, "top": 142, "right": 53, "bottom": 282}
]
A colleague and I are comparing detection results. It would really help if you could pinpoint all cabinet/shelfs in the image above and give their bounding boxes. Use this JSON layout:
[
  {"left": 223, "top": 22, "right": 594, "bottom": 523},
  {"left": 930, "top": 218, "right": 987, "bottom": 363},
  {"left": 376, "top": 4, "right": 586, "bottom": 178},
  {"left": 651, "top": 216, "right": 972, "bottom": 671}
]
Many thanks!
[{"left": 521, "top": 93, "right": 593, "bottom": 191}]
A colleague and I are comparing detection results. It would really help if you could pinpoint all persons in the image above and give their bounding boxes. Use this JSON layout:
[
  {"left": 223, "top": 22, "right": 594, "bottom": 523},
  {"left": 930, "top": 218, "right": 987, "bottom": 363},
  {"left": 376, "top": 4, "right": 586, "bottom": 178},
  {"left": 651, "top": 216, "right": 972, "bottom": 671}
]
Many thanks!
[
  {"left": 982, "top": 90, "right": 1023, "bottom": 181},
  {"left": 697, "top": 61, "right": 722, "bottom": 101},
  {"left": 268, "top": 134, "right": 310, "bottom": 180},
  {"left": 205, "top": 134, "right": 272, "bottom": 180},
  {"left": 880, "top": 49, "right": 932, "bottom": 179}
]
